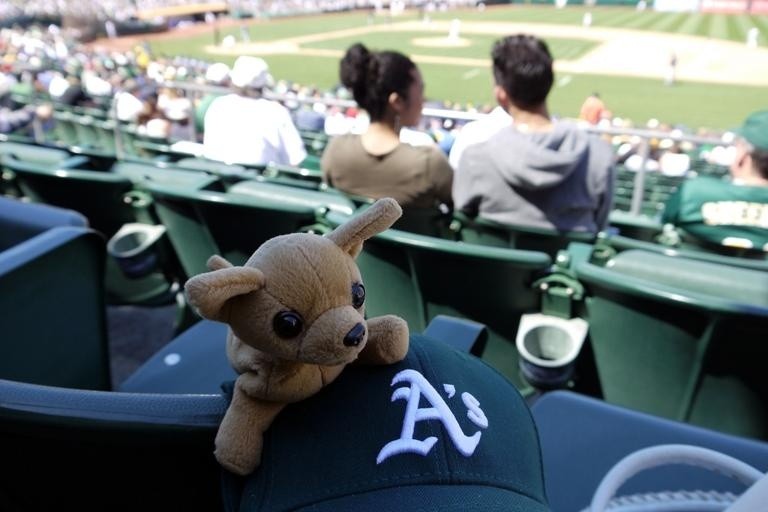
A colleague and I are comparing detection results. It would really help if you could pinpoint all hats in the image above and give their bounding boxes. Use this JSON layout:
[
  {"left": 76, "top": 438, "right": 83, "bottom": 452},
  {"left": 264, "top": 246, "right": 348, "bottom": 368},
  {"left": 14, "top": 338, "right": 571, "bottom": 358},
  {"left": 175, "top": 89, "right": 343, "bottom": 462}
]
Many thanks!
[
  {"left": 206, "top": 56, "right": 352, "bottom": 111},
  {"left": 0, "top": 37, "right": 201, "bottom": 121},
  {"left": 728, "top": 109, "right": 768, "bottom": 149},
  {"left": 219, "top": 335, "right": 552, "bottom": 511}
]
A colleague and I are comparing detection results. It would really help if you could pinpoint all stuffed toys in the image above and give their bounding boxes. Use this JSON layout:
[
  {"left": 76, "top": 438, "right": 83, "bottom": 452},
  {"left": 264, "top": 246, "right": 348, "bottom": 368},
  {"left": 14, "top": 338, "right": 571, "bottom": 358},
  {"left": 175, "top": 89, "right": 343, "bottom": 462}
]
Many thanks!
[{"left": 182, "top": 196, "right": 410, "bottom": 477}]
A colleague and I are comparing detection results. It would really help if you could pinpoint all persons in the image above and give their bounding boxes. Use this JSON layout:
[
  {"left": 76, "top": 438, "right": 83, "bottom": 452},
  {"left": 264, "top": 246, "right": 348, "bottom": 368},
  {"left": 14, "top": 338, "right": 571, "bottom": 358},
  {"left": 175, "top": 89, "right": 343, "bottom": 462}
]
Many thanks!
[
  {"left": 319, "top": 41, "right": 453, "bottom": 210},
  {"left": 450, "top": 32, "right": 615, "bottom": 234},
  {"left": 0, "top": 0, "right": 767, "bottom": 178},
  {"left": 657, "top": 110, "right": 767, "bottom": 252}
]
